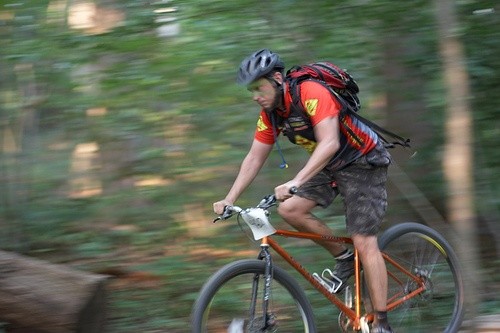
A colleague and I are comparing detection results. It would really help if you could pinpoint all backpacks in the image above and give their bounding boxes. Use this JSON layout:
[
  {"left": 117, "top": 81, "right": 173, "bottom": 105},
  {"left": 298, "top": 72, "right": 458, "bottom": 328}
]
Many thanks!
[{"left": 274, "top": 61, "right": 361, "bottom": 146}]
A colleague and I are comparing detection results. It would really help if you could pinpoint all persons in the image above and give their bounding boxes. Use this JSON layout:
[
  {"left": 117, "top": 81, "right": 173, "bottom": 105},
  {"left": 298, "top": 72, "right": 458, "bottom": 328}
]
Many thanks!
[{"left": 213, "top": 47, "right": 392, "bottom": 333}]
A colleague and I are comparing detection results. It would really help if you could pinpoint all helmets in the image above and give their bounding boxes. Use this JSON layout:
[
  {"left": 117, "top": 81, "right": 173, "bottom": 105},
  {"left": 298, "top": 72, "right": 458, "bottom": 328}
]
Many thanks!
[{"left": 237, "top": 48, "right": 285, "bottom": 87}]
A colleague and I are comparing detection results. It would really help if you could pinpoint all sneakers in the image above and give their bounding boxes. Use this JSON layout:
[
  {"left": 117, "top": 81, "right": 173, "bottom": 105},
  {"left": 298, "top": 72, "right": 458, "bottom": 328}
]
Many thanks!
[
  {"left": 329, "top": 251, "right": 363, "bottom": 293},
  {"left": 371, "top": 325, "right": 396, "bottom": 333}
]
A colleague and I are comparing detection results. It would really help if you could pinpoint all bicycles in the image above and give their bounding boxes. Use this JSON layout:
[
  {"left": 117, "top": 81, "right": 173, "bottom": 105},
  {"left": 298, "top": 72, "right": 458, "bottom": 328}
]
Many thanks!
[{"left": 189, "top": 186, "right": 465, "bottom": 333}]
